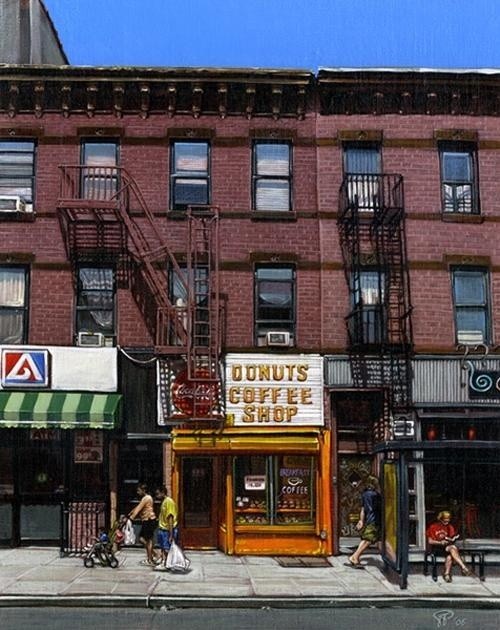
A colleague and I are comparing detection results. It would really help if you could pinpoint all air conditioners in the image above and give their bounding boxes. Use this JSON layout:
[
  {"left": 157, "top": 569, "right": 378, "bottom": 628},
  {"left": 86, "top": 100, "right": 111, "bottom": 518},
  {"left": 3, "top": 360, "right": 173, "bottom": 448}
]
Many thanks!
[
  {"left": 394, "top": 420, "right": 415, "bottom": 440},
  {"left": 0, "top": 195, "right": 26, "bottom": 214},
  {"left": 266, "top": 332, "right": 290, "bottom": 348},
  {"left": 77, "top": 331, "right": 106, "bottom": 347}
]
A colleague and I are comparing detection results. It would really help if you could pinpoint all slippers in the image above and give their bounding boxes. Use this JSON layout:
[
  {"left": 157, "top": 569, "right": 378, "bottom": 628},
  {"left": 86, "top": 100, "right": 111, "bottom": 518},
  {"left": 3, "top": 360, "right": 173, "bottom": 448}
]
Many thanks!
[{"left": 344, "top": 563, "right": 365, "bottom": 569}]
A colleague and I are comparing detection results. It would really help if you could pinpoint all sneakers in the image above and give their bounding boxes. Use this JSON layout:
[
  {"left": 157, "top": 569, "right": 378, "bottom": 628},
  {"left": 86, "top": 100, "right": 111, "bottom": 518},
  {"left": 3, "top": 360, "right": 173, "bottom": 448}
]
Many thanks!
[
  {"left": 185, "top": 558, "right": 191, "bottom": 571},
  {"left": 443, "top": 573, "right": 452, "bottom": 583},
  {"left": 461, "top": 567, "right": 472, "bottom": 576},
  {"left": 140, "top": 553, "right": 168, "bottom": 571}
]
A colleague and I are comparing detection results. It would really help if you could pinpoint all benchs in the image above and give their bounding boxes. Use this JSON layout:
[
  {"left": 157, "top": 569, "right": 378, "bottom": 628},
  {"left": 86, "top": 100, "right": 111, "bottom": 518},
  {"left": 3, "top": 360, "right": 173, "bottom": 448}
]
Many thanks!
[{"left": 424, "top": 544, "right": 499, "bottom": 580}]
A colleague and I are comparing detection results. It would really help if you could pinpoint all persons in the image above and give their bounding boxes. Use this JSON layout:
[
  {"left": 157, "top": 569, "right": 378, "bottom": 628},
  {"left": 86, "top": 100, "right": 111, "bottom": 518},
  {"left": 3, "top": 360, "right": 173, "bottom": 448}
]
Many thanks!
[
  {"left": 428, "top": 510, "right": 472, "bottom": 581},
  {"left": 124, "top": 482, "right": 161, "bottom": 566},
  {"left": 152, "top": 486, "right": 179, "bottom": 572},
  {"left": 349, "top": 475, "right": 382, "bottom": 568},
  {"left": 83, "top": 527, "right": 109, "bottom": 554}
]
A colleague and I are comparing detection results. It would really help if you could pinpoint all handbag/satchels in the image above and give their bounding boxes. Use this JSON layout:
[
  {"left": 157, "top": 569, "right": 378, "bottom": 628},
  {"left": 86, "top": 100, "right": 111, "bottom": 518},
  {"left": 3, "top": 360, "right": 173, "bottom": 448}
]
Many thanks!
[
  {"left": 166, "top": 540, "right": 186, "bottom": 572},
  {"left": 122, "top": 520, "right": 136, "bottom": 545}
]
